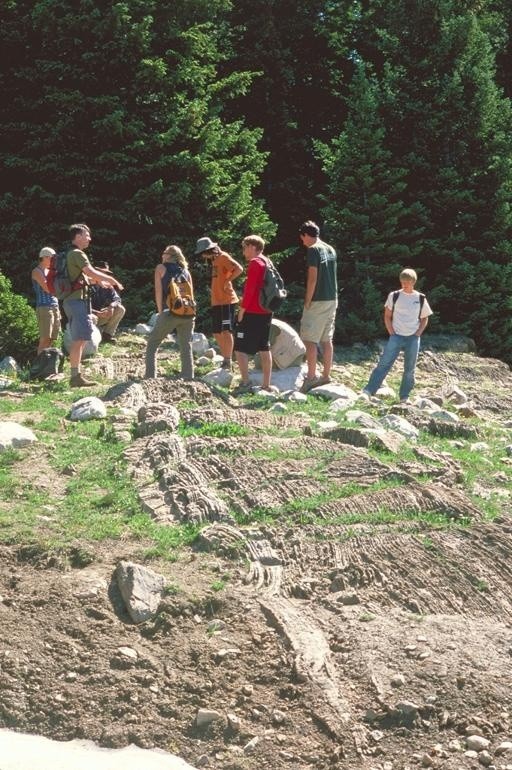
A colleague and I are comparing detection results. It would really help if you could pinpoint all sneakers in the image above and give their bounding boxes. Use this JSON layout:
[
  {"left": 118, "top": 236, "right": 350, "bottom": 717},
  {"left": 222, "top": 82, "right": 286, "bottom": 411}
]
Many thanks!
[
  {"left": 233, "top": 380, "right": 257, "bottom": 395},
  {"left": 299, "top": 374, "right": 333, "bottom": 394},
  {"left": 400, "top": 398, "right": 413, "bottom": 405},
  {"left": 359, "top": 393, "right": 369, "bottom": 401},
  {"left": 69, "top": 371, "right": 97, "bottom": 387},
  {"left": 217, "top": 361, "right": 232, "bottom": 371},
  {"left": 101, "top": 336, "right": 116, "bottom": 342}
]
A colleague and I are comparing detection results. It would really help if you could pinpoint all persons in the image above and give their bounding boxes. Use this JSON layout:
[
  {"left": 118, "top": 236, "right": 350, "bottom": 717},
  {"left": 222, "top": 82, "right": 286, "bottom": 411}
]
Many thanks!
[
  {"left": 31, "top": 246, "right": 63, "bottom": 355},
  {"left": 144, "top": 245, "right": 197, "bottom": 379},
  {"left": 89, "top": 259, "right": 126, "bottom": 345},
  {"left": 361, "top": 267, "right": 434, "bottom": 403},
  {"left": 298, "top": 220, "right": 342, "bottom": 392},
  {"left": 48, "top": 223, "right": 126, "bottom": 387},
  {"left": 235, "top": 235, "right": 285, "bottom": 392},
  {"left": 195, "top": 236, "right": 244, "bottom": 372}
]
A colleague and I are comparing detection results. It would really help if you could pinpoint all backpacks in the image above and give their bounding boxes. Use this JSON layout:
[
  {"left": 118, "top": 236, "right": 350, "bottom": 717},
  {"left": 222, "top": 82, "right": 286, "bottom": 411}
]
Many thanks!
[
  {"left": 167, "top": 272, "right": 197, "bottom": 317},
  {"left": 46, "top": 250, "right": 72, "bottom": 300},
  {"left": 255, "top": 255, "right": 287, "bottom": 313}
]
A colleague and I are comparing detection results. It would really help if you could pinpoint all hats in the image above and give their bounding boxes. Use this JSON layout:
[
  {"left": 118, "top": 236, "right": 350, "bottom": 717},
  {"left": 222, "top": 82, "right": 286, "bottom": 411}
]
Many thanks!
[
  {"left": 38, "top": 247, "right": 56, "bottom": 258},
  {"left": 94, "top": 261, "right": 113, "bottom": 274},
  {"left": 194, "top": 237, "right": 218, "bottom": 255}
]
